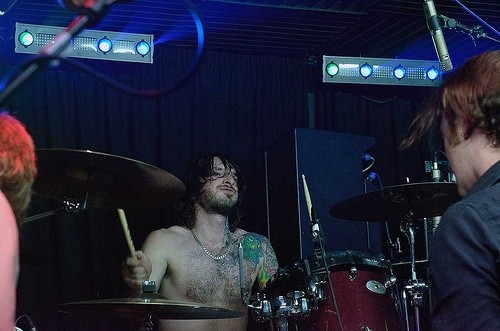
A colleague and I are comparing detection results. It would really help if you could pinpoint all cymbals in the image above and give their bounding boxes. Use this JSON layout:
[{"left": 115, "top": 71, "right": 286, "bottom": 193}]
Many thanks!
[
  {"left": 54, "top": 293, "right": 246, "bottom": 320},
  {"left": 329, "top": 182, "right": 463, "bottom": 221},
  {"left": 31, "top": 148, "right": 187, "bottom": 212}
]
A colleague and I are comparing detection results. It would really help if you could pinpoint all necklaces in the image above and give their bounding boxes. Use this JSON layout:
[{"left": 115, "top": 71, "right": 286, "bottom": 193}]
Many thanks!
[{"left": 190, "top": 223, "right": 231, "bottom": 259}]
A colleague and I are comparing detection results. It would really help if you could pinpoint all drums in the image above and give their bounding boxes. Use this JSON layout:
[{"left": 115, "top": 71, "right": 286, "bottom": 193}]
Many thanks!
[{"left": 260, "top": 251, "right": 405, "bottom": 331}]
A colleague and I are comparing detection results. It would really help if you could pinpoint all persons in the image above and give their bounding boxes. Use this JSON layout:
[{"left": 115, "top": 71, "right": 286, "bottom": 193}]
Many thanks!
[
  {"left": 425, "top": 48, "right": 500, "bottom": 331},
  {"left": 122, "top": 151, "right": 291, "bottom": 331},
  {"left": 0, "top": 112, "right": 38, "bottom": 331}
]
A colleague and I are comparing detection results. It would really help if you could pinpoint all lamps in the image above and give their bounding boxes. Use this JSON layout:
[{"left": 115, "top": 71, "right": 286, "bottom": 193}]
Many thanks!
[
  {"left": 322, "top": 55, "right": 442, "bottom": 87},
  {"left": 14, "top": 23, "right": 155, "bottom": 65}
]
